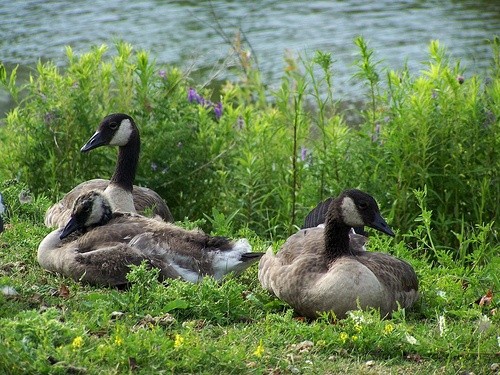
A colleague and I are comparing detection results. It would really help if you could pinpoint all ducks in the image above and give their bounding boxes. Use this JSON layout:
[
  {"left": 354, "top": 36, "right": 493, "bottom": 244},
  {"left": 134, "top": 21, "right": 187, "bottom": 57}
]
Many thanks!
[
  {"left": 37, "top": 111, "right": 266, "bottom": 288},
  {"left": 258, "top": 189, "right": 420, "bottom": 321}
]
